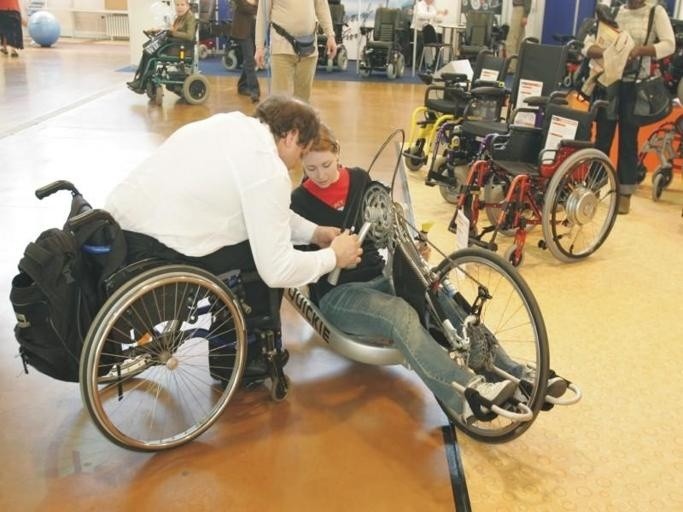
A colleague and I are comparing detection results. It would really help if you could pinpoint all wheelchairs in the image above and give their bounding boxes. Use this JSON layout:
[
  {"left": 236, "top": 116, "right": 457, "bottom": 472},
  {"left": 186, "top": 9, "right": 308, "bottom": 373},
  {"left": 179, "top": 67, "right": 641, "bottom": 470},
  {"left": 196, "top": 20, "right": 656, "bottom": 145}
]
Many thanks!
[
  {"left": 127, "top": 20, "right": 212, "bottom": 106},
  {"left": 402, "top": 44, "right": 518, "bottom": 171},
  {"left": 425, "top": 38, "right": 583, "bottom": 205},
  {"left": 359, "top": 8, "right": 510, "bottom": 80},
  {"left": 563, "top": 19, "right": 682, "bottom": 91},
  {"left": 35, "top": 180, "right": 290, "bottom": 452},
  {"left": 447, "top": 90, "right": 620, "bottom": 269},
  {"left": 197, "top": 0, "right": 349, "bottom": 71},
  {"left": 635, "top": 115, "right": 682, "bottom": 200}
]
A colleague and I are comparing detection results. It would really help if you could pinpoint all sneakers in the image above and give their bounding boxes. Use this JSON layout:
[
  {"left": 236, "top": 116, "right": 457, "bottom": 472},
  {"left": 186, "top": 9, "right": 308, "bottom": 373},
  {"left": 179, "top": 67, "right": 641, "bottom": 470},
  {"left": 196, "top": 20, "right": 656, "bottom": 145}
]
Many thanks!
[
  {"left": 126, "top": 79, "right": 145, "bottom": 89},
  {"left": 462, "top": 377, "right": 517, "bottom": 427},
  {"left": 236, "top": 86, "right": 260, "bottom": 102},
  {"left": 0, "top": 47, "right": 18, "bottom": 58},
  {"left": 617, "top": 193, "right": 631, "bottom": 215},
  {"left": 526, "top": 378, "right": 569, "bottom": 412}
]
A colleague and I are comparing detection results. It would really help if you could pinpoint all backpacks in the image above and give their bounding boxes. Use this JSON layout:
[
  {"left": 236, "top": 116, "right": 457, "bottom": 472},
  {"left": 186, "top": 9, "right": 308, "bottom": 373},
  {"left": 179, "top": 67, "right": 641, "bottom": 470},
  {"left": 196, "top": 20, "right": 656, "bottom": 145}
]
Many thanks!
[{"left": 9, "top": 197, "right": 127, "bottom": 383}]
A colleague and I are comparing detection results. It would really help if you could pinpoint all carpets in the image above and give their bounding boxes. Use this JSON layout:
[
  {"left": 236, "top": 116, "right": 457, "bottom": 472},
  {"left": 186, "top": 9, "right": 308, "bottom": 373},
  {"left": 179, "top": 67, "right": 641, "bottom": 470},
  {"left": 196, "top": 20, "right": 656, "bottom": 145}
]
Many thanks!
[{"left": 113, "top": 54, "right": 516, "bottom": 89}]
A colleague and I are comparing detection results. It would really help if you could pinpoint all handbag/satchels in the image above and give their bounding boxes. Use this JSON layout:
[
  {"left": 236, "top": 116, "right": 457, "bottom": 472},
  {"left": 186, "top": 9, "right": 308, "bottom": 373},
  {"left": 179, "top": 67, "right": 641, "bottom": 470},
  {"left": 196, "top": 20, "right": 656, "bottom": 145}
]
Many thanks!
[
  {"left": 293, "top": 35, "right": 316, "bottom": 56},
  {"left": 623, "top": 78, "right": 674, "bottom": 128},
  {"left": 142, "top": 31, "right": 168, "bottom": 56}
]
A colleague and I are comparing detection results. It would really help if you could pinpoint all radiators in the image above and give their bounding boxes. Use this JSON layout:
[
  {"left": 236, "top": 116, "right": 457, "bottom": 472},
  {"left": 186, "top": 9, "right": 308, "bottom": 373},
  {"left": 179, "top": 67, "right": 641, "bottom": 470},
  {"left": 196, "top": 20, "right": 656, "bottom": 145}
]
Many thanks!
[{"left": 107, "top": 12, "right": 129, "bottom": 40}]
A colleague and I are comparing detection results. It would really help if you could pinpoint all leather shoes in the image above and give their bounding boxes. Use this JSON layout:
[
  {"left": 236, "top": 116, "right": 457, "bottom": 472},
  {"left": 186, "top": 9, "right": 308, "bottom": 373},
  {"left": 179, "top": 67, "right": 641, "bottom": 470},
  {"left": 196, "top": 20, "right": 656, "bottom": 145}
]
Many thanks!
[{"left": 210, "top": 348, "right": 290, "bottom": 381}]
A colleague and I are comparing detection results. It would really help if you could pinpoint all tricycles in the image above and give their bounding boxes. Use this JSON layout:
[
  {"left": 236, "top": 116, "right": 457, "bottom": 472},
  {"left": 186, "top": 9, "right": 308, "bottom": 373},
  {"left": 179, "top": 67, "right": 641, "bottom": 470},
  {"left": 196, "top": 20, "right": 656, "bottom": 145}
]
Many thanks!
[{"left": 284, "top": 129, "right": 581, "bottom": 443}]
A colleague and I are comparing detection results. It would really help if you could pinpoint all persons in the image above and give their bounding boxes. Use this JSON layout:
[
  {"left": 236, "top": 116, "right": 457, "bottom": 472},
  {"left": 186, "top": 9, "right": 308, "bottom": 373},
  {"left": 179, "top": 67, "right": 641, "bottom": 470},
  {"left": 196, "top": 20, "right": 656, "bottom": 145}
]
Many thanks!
[
  {"left": 232, "top": 0, "right": 260, "bottom": 102},
  {"left": 292, "top": 124, "right": 568, "bottom": 421},
  {"left": 580, "top": 1, "right": 676, "bottom": 214},
  {"left": 98, "top": 93, "right": 318, "bottom": 390},
  {"left": 127, "top": 0, "right": 198, "bottom": 90},
  {"left": 410, "top": 1, "right": 441, "bottom": 65},
  {"left": 0, "top": 0, "right": 23, "bottom": 57},
  {"left": 254, "top": 0, "right": 338, "bottom": 103}
]
